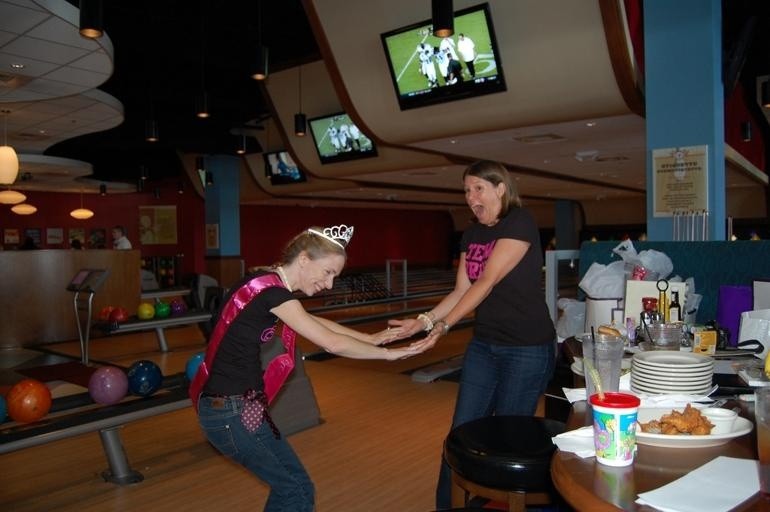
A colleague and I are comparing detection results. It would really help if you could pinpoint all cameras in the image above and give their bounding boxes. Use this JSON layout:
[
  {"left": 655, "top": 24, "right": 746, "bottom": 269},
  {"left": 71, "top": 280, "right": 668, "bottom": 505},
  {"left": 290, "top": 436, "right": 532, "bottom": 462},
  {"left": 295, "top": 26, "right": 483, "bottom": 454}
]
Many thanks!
[{"left": 703, "top": 320, "right": 728, "bottom": 350}]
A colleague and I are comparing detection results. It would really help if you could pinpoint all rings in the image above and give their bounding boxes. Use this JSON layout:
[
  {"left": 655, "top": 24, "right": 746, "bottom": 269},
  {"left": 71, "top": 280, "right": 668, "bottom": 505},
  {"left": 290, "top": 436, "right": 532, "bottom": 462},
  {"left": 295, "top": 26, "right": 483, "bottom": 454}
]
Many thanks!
[{"left": 387, "top": 326, "right": 390, "bottom": 331}]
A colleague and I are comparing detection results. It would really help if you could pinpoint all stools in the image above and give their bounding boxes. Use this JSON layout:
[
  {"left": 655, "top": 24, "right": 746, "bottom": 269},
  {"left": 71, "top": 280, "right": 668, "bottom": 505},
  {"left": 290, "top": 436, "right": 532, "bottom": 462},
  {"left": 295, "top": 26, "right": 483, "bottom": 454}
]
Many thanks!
[{"left": 438, "top": 416, "right": 566, "bottom": 511}]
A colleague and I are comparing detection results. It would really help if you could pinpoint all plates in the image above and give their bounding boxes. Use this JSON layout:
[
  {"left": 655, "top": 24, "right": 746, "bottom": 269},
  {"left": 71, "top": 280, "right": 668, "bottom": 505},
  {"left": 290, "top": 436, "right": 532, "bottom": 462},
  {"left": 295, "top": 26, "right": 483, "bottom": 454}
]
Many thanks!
[
  {"left": 628, "top": 349, "right": 718, "bottom": 397},
  {"left": 633, "top": 405, "right": 757, "bottom": 451}
]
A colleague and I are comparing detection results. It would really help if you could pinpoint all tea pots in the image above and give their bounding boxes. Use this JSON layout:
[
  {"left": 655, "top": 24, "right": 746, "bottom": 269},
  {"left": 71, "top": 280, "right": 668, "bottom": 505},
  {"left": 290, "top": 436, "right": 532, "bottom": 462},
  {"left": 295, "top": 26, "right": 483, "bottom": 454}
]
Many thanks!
[{"left": 638, "top": 323, "right": 680, "bottom": 350}]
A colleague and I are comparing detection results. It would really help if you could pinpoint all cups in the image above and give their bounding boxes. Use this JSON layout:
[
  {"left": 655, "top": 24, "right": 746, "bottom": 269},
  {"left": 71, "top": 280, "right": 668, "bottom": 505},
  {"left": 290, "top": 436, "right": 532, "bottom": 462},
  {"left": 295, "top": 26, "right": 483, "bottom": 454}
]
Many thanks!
[
  {"left": 593, "top": 460, "right": 636, "bottom": 512},
  {"left": 699, "top": 407, "right": 739, "bottom": 432},
  {"left": 582, "top": 333, "right": 626, "bottom": 408},
  {"left": 753, "top": 386, "right": 770, "bottom": 467},
  {"left": 587, "top": 390, "right": 640, "bottom": 466}
]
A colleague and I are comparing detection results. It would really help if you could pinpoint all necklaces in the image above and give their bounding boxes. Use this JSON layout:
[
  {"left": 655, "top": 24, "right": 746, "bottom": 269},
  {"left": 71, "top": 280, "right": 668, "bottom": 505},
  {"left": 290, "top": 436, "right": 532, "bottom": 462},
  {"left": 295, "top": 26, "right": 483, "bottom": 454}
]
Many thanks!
[{"left": 278, "top": 266, "right": 293, "bottom": 292}]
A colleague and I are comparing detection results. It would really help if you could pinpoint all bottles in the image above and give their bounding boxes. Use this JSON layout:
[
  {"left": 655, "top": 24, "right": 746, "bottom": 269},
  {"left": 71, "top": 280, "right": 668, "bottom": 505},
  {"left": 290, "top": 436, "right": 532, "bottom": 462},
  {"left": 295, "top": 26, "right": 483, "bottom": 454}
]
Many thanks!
[
  {"left": 651, "top": 307, "right": 662, "bottom": 325},
  {"left": 639, "top": 310, "right": 652, "bottom": 327},
  {"left": 669, "top": 286, "right": 681, "bottom": 323}
]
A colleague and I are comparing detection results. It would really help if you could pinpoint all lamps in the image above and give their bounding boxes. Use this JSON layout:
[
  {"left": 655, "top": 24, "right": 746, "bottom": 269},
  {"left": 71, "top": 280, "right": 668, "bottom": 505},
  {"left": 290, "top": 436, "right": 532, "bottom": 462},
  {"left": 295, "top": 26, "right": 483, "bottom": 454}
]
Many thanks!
[
  {"left": 292, "top": 51, "right": 308, "bottom": 138},
  {"left": 78, "top": 2, "right": 105, "bottom": 40},
  {"left": 171, "top": 146, "right": 217, "bottom": 197},
  {"left": 235, "top": 104, "right": 249, "bottom": 156},
  {"left": 196, "top": 57, "right": 215, "bottom": 119},
  {"left": 249, "top": 7, "right": 270, "bottom": 82},
  {"left": 430, "top": 0, "right": 454, "bottom": 38},
  {"left": 1, "top": 59, "right": 165, "bottom": 223}
]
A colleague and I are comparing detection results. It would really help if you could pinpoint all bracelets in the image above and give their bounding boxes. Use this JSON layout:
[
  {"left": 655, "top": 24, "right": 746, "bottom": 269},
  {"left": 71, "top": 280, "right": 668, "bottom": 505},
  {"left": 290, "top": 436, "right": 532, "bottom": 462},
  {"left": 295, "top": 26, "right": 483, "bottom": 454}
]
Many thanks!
[
  {"left": 439, "top": 321, "right": 449, "bottom": 335},
  {"left": 417, "top": 314, "right": 433, "bottom": 331}
]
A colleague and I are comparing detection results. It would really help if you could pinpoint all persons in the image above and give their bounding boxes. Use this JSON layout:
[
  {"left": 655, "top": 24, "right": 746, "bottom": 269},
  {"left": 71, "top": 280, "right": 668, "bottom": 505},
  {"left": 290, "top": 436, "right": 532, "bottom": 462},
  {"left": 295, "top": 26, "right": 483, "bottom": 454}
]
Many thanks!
[
  {"left": 327, "top": 122, "right": 361, "bottom": 153},
  {"left": 277, "top": 158, "right": 291, "bottom": 174},
  {"left": 112, "top": 225, "right": 132, "bottom": 249},
  {"left": 383, "top": 158, "right": 557, "bottom": 512},
  {"left": 190, "top": 225, "right": 428, "bottom": 512},
  {"left": 417, "top": 33, "right": 477, "bottom": 87}
]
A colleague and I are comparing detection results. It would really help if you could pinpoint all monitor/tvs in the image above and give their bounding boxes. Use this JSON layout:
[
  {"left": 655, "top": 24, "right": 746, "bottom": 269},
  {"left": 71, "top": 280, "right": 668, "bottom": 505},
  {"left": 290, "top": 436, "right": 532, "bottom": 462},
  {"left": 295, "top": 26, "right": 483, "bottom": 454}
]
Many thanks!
[
  {"left": 307, "top": 111, "right": 379, "bottom": 165},
  {"left": 379, "top": 1, "right": 508, "bottom": 112},
  {"left": 262, "top": 150, "right": 307, "bottom": 186}
]
